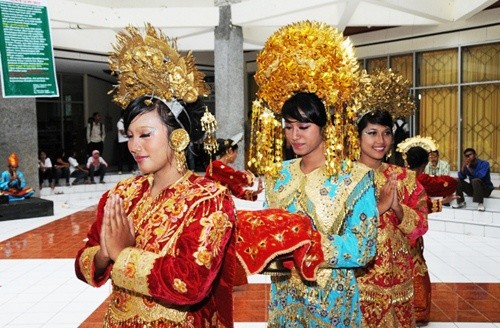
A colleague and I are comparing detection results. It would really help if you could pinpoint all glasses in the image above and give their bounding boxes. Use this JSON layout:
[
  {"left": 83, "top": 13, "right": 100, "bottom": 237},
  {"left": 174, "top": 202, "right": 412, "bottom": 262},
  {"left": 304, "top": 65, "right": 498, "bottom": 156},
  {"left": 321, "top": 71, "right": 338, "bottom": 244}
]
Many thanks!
[{"left": 465, "top": 153, "right": 472, "bottom": 158}]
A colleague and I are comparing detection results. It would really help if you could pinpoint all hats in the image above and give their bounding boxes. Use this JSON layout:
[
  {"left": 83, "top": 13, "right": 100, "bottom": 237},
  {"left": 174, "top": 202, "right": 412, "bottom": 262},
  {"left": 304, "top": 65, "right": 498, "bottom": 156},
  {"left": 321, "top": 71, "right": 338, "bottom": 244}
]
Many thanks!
[
  {"left": 93, "top": 150, "right": 99, "bottom": 156},
  {"left": 8, "top": 153, "right": 18, "bottom": 167}
]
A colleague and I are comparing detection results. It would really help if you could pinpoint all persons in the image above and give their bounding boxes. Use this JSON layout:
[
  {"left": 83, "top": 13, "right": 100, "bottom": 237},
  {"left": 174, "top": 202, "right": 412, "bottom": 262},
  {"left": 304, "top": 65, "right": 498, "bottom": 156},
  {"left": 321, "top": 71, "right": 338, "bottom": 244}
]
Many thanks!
[
  {"left": 389, "top": 146, "right": 495, "bottom": 211},
  {"left": 116, "top": 109, "right": 137, "bottom": 176},
  {"left": 74, "top": 90, "right": 238, "bottom": 328},
  {"left": 85, "top": 112, "right": 106, "bottom": 155},
  {"left": 36, "top": 150, "right": 109, "bottom": 186},
  {"left": 0, "top": 151, "right": 36, "bottom": 202},
  {"left": 356, "top": 109, "right": 433, "bottom": 328},
  {"left": 264, "top": 89, "right": 379, "bottom": 328},
  {"left": 203, "top": 137, "right": 264, "bottom": 202}
]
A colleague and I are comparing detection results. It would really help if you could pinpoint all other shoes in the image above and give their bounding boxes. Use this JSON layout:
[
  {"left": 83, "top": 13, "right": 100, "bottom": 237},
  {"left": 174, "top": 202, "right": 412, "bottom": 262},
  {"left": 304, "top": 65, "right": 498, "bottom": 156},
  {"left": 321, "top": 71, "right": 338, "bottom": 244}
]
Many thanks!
[
  {"left": 118, "top": 171, "right": 122, "bottom": 174},
  {"left": 39, "top": 186, "right": 42, "bottom": 189},
  {"left": 54, "top": 184, "right": 59, "bottom": 186},
  {"left": 85, "top": 180, "right": 90, "bottom": 184},
  {"left": 91, "top": 181, "right": 96, "bottom": 184},
  {"left": 72, "top": 182, "right": 79, "bottom": 185},
  {"left": 49, "top": 183, "right": 54, "bottom": 187},
  {"left": 442, "top": 203, "right": 450, "bottom": 206},
  {"left": 478, "top": 205, "right": 485, "bottom": 211},
  {"left": 66, "top": 183, "right": 70, "bottom": 186},
  {"left": 452, "top": 202, "right": 466, "bottom": 208},
  {"left": 131, "top": 171, "right": 136, "bottom": 174},
  {"left": 100, "top": 181, "right": 105, "bottom": 183}
]
